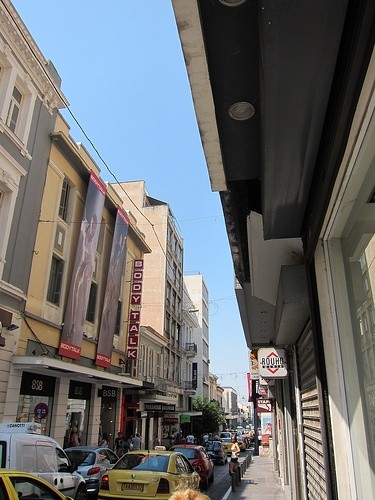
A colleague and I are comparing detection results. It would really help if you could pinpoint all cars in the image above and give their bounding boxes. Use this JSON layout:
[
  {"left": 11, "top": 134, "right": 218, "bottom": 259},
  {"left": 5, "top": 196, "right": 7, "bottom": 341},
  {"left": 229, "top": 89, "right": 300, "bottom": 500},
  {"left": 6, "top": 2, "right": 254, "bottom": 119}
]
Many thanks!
[
  {"left": 98, "top": 446, "right": 202, "bottom": 500},
  {"left": 0, "top": 468, "right": 75, "bottom": 500},
  {"left": 201, "top": 441, "right": 228, "bottom": 465},
  {"left": 219, "top": 424, "right": 255, "bottom": 455},
  {"left": 63, "top": 446, "right": 120, "bottom": 495},
  {"left": 168, "top": 444, "right": 215, "bottom": 490}
]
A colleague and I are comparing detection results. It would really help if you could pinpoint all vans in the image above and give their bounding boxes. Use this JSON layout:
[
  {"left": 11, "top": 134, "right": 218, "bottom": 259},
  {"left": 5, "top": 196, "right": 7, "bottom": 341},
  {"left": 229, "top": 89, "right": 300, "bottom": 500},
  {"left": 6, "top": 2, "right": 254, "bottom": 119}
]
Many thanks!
[{"left": 0, "top": 422, "right": 87, "bottom": 500}]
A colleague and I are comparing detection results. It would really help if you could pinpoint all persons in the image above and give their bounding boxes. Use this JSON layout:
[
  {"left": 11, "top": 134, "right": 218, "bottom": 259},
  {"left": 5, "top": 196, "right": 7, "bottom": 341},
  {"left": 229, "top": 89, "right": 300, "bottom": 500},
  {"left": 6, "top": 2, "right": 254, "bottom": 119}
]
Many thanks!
[{"left": 69, "top": 419, "right": 255, "bottom": 475}]
